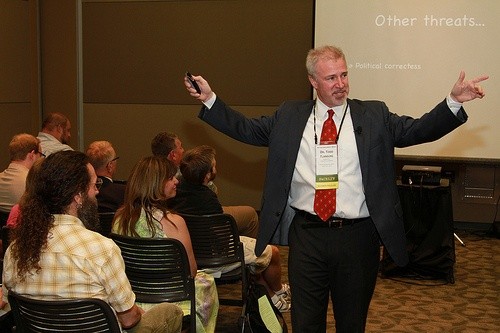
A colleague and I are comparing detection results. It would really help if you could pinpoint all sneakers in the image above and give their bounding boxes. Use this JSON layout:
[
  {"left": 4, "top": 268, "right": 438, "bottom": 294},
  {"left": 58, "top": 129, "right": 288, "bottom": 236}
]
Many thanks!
[
  {"left": 275, "top": 283, "right": 291, "bottom": 298},
  {"left": 275, "top": 299, "right": 291, "bottom": 312}
]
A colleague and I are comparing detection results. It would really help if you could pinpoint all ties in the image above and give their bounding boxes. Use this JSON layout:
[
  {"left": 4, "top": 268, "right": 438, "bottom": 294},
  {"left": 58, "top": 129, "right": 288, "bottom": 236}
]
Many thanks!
[{"left": 314, "top": 110, "right": 338, "bottom": 222}]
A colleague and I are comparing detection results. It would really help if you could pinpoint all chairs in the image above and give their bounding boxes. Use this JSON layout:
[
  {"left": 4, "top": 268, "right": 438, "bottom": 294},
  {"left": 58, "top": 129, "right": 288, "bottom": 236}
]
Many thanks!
[{"left": 0, "top": 178, "right": 254, "bottom": 333}]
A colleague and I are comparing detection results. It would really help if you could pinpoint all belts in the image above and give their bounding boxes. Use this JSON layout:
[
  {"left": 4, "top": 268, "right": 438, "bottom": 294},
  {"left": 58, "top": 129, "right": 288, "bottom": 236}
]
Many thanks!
[{"left": 295, "top": 211, "right": 370, "bottom": 228}]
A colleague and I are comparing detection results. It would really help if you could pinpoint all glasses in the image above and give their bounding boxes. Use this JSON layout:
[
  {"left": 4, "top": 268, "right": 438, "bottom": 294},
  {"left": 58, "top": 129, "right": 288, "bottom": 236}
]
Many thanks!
[
  {"left": 88, "top": 178, "right": 103, "bottom": 190},
  {"left": 38, "top": 151, "right": 46, "bottom": 159},
  {"left": 106, "top": 155, "right": 119, "bottom": 168}
]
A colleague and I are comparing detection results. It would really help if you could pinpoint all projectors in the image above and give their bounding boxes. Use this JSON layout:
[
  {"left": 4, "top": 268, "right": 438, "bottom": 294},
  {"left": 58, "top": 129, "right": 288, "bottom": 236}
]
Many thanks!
[{"left": 402, "top": 163, "right": 442, "bottom": 186}]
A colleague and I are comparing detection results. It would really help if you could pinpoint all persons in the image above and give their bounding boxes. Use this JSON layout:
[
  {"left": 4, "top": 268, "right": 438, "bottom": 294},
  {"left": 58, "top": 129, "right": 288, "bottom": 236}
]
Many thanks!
[
  {"left": 147, "top": 131, "right": 261, "bottom": 242},
  {"left": 0, "top": 286, "right": 16, "bottom": 333},
  {"left": 80, "top": 140, "right": 133, "bottom": 233},
  {"left": 183, "top": 45, "right": 489, "bottom": 333},
  {"left": 28, "top": 110, "right": 76, "bottom": 163},
  {"left": 8, "top": 156, "right": 43, "bottom": 231},
  {"left": 3, "top": 150, "right": 183, "bottom": 333},
  {"left": 165, "top": 146, "right": 295, "bottom": 313},
  {"left": 112, "top": 155, "right": 218, "bottom": 333},
  {"left": 0, "top": 132, "right": 44, "bottom": 220}
]
